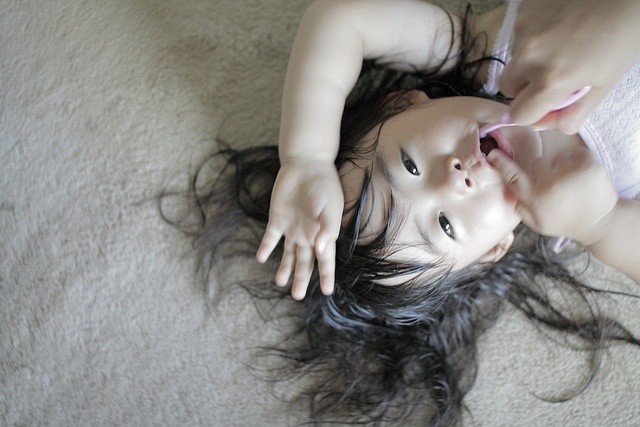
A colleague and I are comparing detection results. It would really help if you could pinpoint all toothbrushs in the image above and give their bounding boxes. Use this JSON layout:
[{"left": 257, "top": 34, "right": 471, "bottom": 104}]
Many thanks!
[{"left": 480, "top": 83, "right": 593, "bottom": 138}]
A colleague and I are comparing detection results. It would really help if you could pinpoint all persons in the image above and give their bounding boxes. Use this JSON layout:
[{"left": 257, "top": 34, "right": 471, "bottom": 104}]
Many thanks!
[
  {"left": 157, "top": 1, "right": 639, "bottom": 427},
  {"left": 499, "top": 2, "right": 639, "bottom": 135}
]
